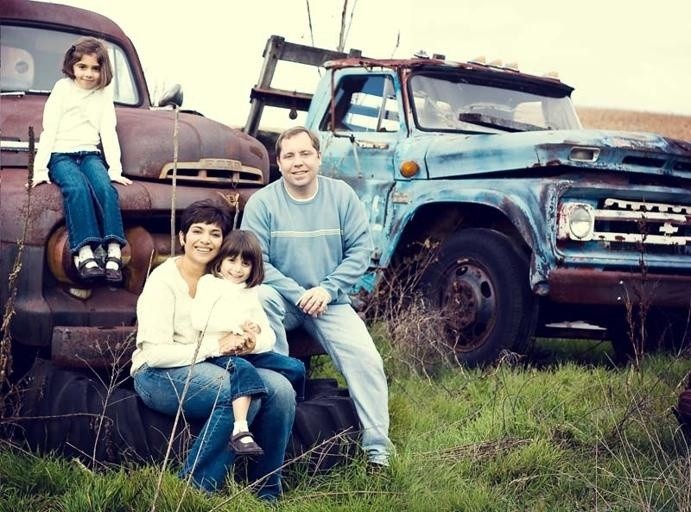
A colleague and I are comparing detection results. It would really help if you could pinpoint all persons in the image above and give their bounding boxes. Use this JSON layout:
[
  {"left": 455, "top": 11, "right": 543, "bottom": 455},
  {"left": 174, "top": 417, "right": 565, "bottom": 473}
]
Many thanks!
[
  {"left": 130, "top": 198, "right": 297, "bottom": 503},
  {"left": 191, "top": 228, "right": 308, "bottom": 455},
  {"left": 25, "top": 34, "right": 134, "bottom": 285},
  {"left": 239, "top": 126, "right": 396, "bottom": 479}
]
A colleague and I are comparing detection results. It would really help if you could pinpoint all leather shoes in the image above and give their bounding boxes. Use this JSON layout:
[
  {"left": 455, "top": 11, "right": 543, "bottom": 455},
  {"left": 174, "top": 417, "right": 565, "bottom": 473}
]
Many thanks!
[
  {"left": 104, "top": 256, "right": 124, "bottom": 283},
  {"left": 229, "top": 432, "right": 263, "bottom": 457},
  {"left": 75, "top": 257, "right": 102, "bottom": 276}
]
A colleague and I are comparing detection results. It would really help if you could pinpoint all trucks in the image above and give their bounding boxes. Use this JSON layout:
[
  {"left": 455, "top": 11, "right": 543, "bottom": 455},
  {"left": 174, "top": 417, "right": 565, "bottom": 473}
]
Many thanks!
[
  {"left": 246, "top": 35, "right": 689, "bottom": 372},
  {"left": 1, "top": 2, "right": 268, "bottom": 462}
]
